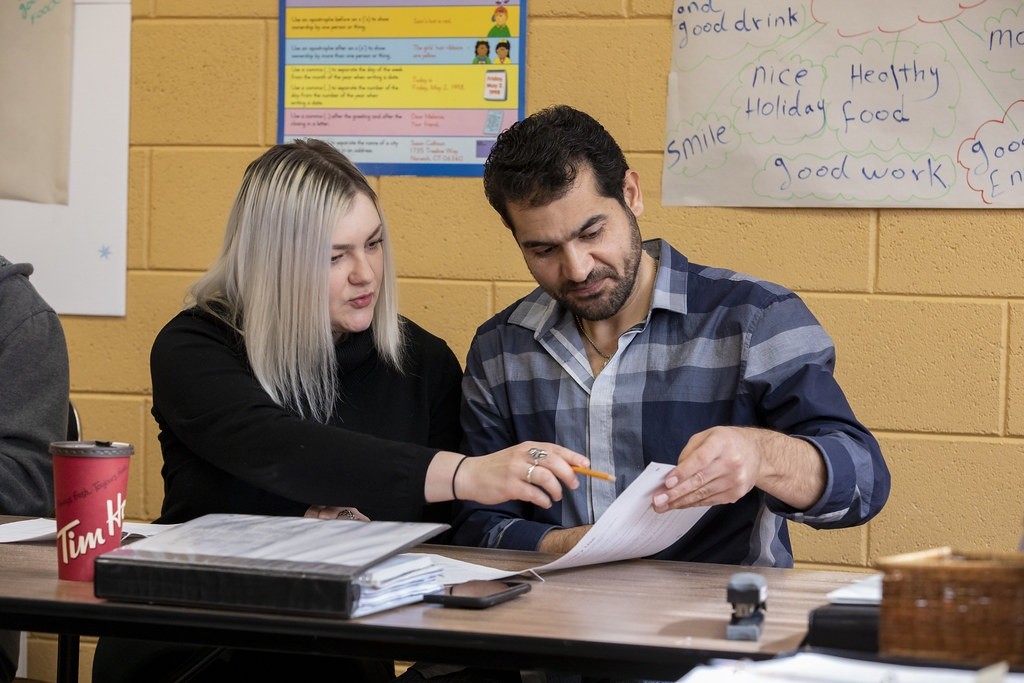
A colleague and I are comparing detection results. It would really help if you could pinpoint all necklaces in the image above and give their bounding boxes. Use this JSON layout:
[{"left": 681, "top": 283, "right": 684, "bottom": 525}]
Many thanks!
[{"left": 576, "top": 310, "right": 615, "bottom": 372}]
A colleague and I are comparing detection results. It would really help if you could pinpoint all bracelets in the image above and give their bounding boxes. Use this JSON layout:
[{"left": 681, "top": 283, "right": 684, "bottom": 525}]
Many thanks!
[{"left": 452, "top": 455, "right": 467, "bottom": 501}]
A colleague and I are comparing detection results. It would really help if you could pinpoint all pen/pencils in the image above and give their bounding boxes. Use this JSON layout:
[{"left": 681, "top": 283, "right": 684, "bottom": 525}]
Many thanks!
[{"left": 572, "top": 466, "right": 616, "bottom": 482}]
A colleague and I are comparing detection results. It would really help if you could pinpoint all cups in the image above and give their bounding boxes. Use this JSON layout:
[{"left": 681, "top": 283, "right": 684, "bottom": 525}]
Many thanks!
[{"left": 49, "top": 441, "right": 134, "bottom": 581}]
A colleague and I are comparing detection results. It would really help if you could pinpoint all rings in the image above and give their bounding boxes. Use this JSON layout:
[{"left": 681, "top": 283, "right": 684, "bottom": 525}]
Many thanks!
[
  {"left": 526, "top": 464, "right": 538, "bottom": 483},
  {"left": 338, "top": 509, "right": 355, "bottom": 519},
  {"left": 527, "top": 448, "right": 548, "bottom": 465}
]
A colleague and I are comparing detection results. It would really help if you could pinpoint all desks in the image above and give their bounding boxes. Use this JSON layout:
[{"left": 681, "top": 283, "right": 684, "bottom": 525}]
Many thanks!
[{"left": 0, "top": 511, "right": 1024, "bottom": 683}]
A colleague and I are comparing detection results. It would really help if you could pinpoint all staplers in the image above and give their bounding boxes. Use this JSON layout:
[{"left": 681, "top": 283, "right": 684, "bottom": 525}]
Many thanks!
[{"left": 727, "top": 570, "right": 768, "bottom": 641}]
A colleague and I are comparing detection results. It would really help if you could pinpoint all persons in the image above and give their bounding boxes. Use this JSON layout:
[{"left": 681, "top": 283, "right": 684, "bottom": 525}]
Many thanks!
[
  {"left": 0, "top": 250, "right": 72, "bottom": 683},
  {"left": 93, "top": 137, "right": 590, "bottom": 683},
  {"left": 448, "top": 105, "right": 891, "bottom": 683}
]
